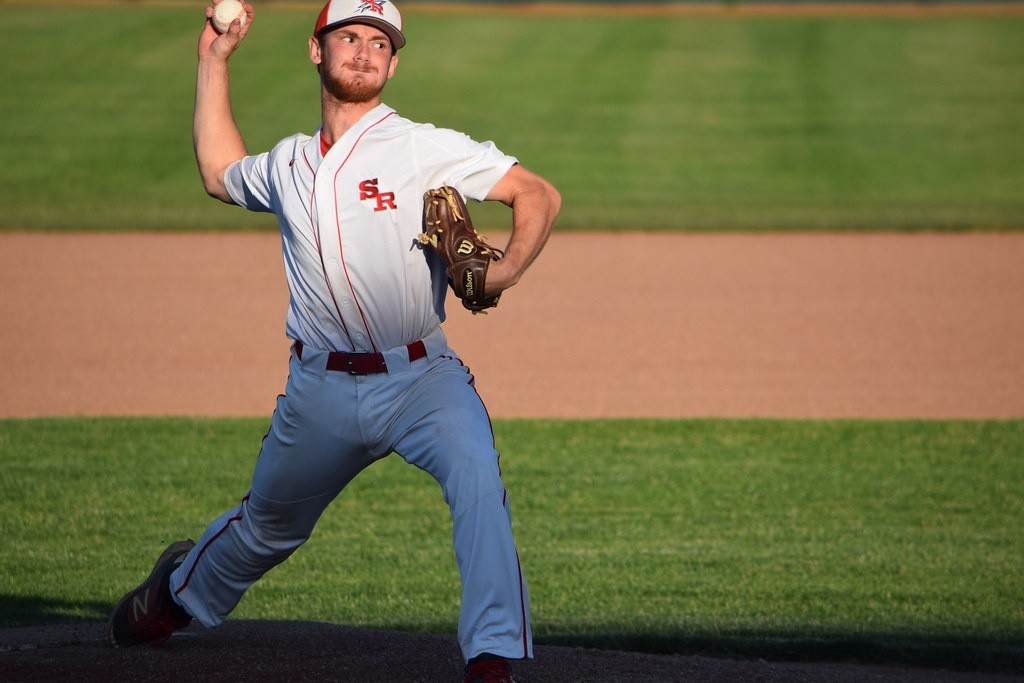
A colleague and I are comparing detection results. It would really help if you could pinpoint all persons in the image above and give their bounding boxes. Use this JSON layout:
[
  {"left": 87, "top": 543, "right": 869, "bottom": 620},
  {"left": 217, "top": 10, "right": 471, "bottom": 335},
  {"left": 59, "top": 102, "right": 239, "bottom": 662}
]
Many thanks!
[{"left": 110, "top": 0, "right": 562, "bottom": 683}]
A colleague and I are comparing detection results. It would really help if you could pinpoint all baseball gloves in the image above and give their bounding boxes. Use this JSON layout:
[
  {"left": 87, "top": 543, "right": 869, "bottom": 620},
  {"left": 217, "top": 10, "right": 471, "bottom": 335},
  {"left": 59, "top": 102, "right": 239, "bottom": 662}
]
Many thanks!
[{"left": 421, "top": 185, "right": 504, "bottom": 312}]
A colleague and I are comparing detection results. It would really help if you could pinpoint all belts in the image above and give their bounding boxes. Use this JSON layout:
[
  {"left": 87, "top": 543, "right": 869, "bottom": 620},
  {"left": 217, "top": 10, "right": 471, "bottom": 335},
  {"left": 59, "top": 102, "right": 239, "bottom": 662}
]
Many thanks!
[{"left": 293, "top": 339, "right": 427, "bottom": 376}]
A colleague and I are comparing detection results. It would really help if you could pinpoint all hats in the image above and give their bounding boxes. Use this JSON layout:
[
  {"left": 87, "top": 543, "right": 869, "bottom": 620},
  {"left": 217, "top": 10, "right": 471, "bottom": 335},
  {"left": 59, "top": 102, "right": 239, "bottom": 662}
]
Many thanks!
[{"left": 313, "top": 0, "right": 406, "bottom": 50}]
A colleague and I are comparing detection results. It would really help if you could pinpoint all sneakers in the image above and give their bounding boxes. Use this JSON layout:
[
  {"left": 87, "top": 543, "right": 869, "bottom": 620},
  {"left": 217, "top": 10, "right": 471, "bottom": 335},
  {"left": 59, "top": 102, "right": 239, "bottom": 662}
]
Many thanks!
[
  {"left": 107, "top": 539, "right": 197, "bottom": 650},
  {"left": 462, "top": 653, "right": 516, "bottom": 683}
]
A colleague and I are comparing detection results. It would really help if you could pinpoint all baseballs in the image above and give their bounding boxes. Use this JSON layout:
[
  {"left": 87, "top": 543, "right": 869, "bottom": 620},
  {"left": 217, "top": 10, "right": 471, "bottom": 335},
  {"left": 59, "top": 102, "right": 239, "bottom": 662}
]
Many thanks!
[{"left": 211, "top": 0, "right": 248, "bottom": 34}]
